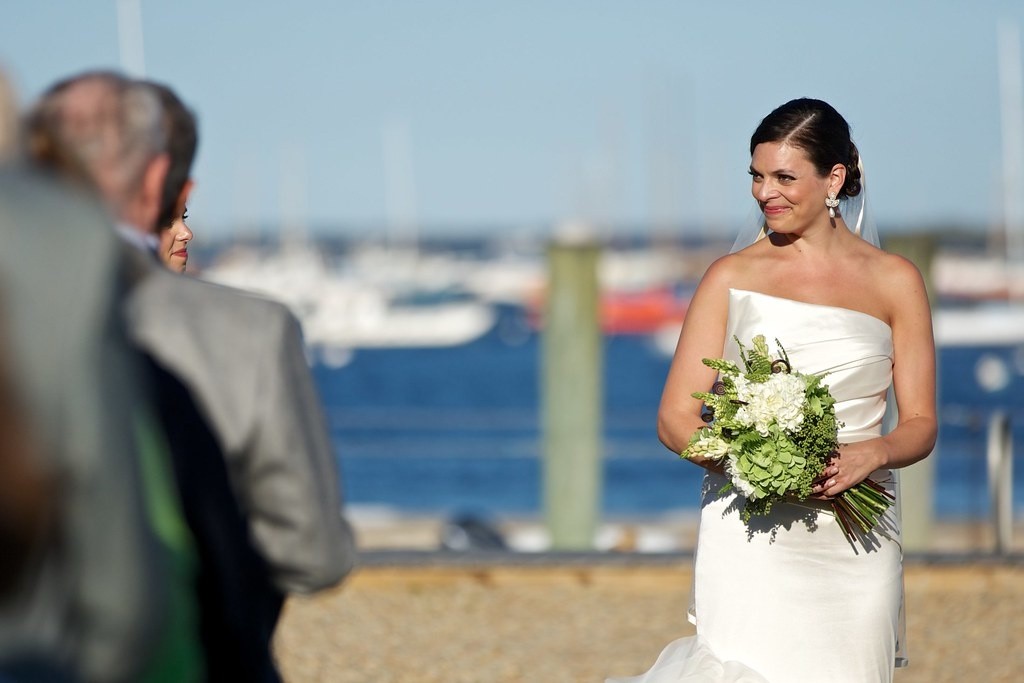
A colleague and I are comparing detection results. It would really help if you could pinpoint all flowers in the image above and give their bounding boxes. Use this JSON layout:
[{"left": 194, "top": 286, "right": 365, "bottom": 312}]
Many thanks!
[{"left": 679, "top": 334, "right": 896, "bottom": 542}]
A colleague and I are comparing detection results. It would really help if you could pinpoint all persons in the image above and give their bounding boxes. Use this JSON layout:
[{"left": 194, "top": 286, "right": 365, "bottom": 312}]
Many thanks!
[
  {"left": 0, "top": 66, "right": 357, "bottom": 683},
  {"left": 600, "top": 96, "right": 939, "bottom": 683},
  {"left": 159, "top": 204, "right": 194, "bottom": 275}
]
